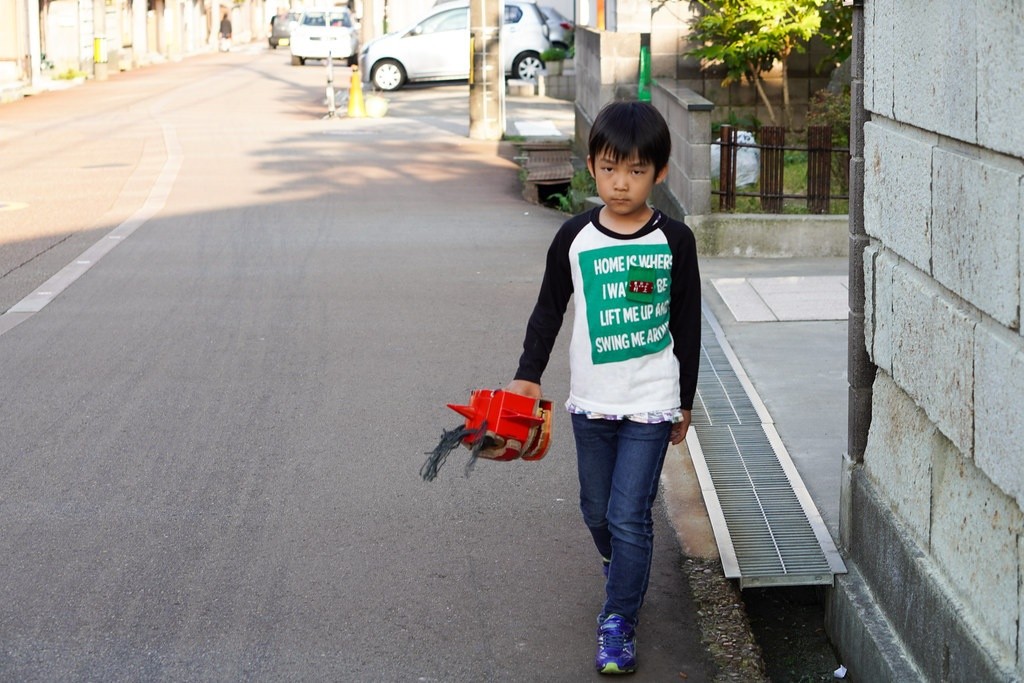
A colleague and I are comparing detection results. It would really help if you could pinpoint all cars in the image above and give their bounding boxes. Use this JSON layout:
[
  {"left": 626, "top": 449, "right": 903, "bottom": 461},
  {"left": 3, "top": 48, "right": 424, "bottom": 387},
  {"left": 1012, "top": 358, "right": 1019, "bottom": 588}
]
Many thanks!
[
  {"left": 538, "top": 4, "right": 576, "bottom": 53},
  {"left": 356, "top": 1, "right": 551, "bottom": 94}
]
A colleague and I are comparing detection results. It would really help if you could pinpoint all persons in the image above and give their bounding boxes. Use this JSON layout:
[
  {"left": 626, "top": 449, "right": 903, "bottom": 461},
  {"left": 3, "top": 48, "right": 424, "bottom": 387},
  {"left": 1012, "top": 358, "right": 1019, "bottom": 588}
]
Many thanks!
[
  {"left": 506, "top": 101, "right": 701, "bottom": 673},
  {"left": 220, "top": 14, "right": 232, "bottom": 52}
]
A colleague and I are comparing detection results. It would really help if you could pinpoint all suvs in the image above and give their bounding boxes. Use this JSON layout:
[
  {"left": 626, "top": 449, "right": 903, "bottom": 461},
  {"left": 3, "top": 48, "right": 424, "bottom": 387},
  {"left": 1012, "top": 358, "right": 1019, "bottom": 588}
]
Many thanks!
[
  {"left": 268, "top": 12, "right": 290, "bottom": 49},
  {"left": 289, "top": 5, "right": 360, "bottom": 69}
]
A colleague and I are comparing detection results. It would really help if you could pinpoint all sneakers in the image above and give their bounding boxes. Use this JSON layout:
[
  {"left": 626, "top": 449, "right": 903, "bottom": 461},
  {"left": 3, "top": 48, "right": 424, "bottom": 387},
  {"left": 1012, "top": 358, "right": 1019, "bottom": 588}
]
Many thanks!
[
  {"left": 595, "top": 614, "right": 638, "bottom": 673},
  {"left": 602, "top": 563, "right": 610, "bottom": 578}
]
